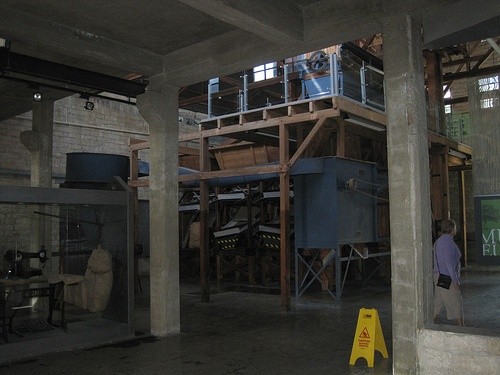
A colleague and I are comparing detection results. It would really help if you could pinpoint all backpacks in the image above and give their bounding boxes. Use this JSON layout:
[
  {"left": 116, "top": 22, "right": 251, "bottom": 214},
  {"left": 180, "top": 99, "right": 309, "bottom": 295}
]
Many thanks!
[{"left": 436, "top": 273, "right": 452, "bottom": 289}]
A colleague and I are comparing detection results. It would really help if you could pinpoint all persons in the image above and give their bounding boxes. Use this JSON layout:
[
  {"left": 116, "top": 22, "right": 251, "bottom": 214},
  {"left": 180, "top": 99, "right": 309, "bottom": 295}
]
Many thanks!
[{"left": 434, "top": 220, "right": 463, "bottom": 326}]
